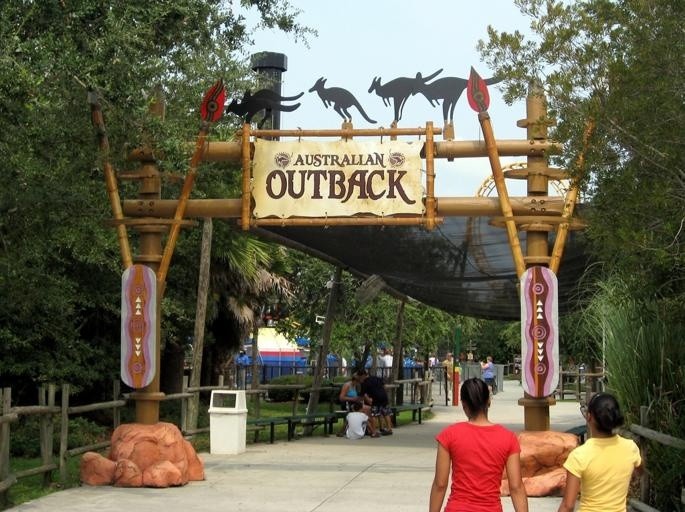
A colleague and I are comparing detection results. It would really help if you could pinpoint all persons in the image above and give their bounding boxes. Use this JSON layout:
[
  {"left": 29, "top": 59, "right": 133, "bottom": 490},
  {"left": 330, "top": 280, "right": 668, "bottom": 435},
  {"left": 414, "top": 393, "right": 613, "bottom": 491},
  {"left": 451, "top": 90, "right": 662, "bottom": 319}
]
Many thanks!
[
  {"left": 558, "top": 392, "right": 644, "bottom": 512},
  {"left": 326, "top": 343, "right": 473, "bottom": 440},
  {"left": 479, "top": 356, "right": 495, "bottom": 399},
  {"left": 429, "top": 376, "right": 528, "bottom": 512}
]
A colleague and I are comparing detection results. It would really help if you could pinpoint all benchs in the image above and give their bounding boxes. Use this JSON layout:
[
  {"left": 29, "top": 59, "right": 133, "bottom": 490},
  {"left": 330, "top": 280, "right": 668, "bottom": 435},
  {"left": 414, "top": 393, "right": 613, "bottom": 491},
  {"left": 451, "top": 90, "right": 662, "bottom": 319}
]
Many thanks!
[{"left": 243, "top": 399, "right": 428, "bottom": 443}]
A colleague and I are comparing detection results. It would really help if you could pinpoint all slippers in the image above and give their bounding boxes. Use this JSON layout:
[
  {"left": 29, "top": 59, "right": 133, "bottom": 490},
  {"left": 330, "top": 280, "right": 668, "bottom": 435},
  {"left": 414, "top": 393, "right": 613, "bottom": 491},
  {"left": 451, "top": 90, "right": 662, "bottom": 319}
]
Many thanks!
[{"left": 370, "top": 429, "right": 392, "bottom": 438}]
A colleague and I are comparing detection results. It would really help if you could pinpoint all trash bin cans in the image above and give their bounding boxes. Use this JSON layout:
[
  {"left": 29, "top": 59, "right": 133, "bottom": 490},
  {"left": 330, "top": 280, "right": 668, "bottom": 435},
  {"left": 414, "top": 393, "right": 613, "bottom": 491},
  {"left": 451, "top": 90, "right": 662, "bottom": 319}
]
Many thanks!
[{"left": 208, "top": 389, "right": 248, "bottom": 454}]
